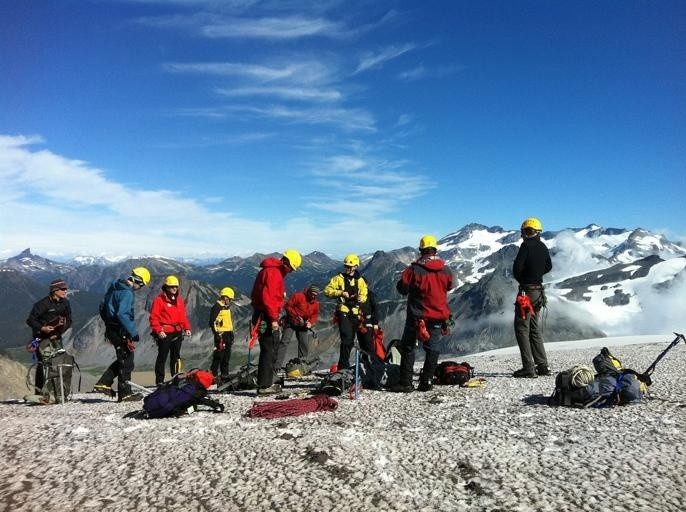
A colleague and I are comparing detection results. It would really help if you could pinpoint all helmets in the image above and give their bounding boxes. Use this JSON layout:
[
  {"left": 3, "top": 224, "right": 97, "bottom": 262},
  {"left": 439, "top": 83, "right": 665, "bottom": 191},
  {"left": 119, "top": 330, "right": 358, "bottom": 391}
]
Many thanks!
[
  {"left": 521, "top": 218, "right": 542, "bottom": 231},
  {"left": 344, "top": 256, "right": 360, "bottom": 267},
  {"left": 221, "top": 287, "right": 234, "bottom": 299},
  {"left": 164, "top": 276, "right": 179, "bottom": 286},
  {"left": 132, "top": 267, "right": 151, "bottom": 285},
  {"left": 283, "top": 250, "right": 302, "bottom": 271},
  {"left": 420, "top": 237, "right": 437, "bottom": 250}
]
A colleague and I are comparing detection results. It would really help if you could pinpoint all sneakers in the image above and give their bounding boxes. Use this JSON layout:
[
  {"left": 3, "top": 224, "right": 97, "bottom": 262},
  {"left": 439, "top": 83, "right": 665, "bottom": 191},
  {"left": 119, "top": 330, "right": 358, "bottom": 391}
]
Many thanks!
[
  {"left": 93, "top": 385, "right": 116, "bottom": 397},
  {"left": 418, "top": 379, "right": 433, "bottom": 391},
  {"left": 258, "top": 384, "right": 282, "bottom": 394},
  {"left": 513, "top": 369, "right": 538, "bottom": 378},
  {"left": 119, "top": 393, "right": 143, "bottom": 403},
  {"left": 535, "top": 366, "right": 551, "bottom": 375},
  {"left": 389, "top": 384, "right": 415, "bottom": 392}
]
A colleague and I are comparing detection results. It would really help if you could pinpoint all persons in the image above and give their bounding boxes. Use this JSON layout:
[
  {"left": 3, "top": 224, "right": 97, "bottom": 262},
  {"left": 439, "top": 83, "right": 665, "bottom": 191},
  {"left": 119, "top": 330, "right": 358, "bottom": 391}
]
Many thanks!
[
  {"left": 512, "top": 218, "right": 551, "bottom": 379},
  {"left": 27, "top": 279, "right": 72, "bottom": 401},
  {"left": 357, "top": 277, "right": 380, "bottom": 367},
  {"left": 251, "top": 250, "right": 302, "bottom": 394},
  {"left": 207, "top": 287, "right": 234, "bottom": 380},
  {"left": 94, "top": 268, "right": 142, "bottom": 401},
  {"left": 396, "top": 234, "right": 456, "bottom": 393},
  {"left": 323, "top": 254, "right": 372, "bottom": 371},
  {"left": 152, "top": 276, "right": 191, "bottom": 386},
  {"left": 273, "top": 284, "right": 320, "bottom": 370}
]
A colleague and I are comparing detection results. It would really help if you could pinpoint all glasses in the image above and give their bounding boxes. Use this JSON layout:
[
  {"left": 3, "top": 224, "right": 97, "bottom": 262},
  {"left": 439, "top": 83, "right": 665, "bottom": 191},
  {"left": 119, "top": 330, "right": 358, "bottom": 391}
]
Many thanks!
[{"left": 128, "top": 277, "right": 144, "bottom": 287}]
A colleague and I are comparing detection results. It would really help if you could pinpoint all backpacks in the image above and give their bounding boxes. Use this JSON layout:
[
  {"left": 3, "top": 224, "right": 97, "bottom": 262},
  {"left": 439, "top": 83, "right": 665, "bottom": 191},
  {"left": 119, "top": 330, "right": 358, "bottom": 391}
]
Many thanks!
[
  {"left": 316, "top": 365, "right": 361, "bottom": 396},
  {"left": 385, "top": 340, "right": 402, "bottom": 365},
  {"left": 213, "top": 363, "right": 258, "bottom": 394},
  {"left": 359, "top": 353, "right": 400, "bottom": 390},
  {"left": 592, "top": 374, "right": 641, "bottom": 408},
  {"left": 286, "top": 357, "right": 319, "bottom": 382},
  {"left": 551, "top": 366, "right": 594, "bottom": 406},
  {"left": 26, "top": 346, "right": 81, "bottom": 404},
  {"left": 593, "top": 348, "right": 652, "bottom": 399},
  {"left": 143, "top": 373, "right": 224, "bottom": 419},
  {"left": 434, "top": 362, "right": 470, "bottom": 385}
]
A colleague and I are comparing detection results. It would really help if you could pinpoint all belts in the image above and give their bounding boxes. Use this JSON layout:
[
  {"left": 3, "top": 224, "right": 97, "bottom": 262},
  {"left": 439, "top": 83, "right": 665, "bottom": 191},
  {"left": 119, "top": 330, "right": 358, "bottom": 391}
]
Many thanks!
[{"left": 519, "top": 285, "right": 542, "bottom": 290}]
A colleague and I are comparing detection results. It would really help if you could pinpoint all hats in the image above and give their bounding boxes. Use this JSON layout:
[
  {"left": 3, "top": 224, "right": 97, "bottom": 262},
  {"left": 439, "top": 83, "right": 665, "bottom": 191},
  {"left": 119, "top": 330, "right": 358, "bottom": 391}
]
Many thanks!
[
  {"left": 50, "top": 279, "right": 68, "bottom": 293},
  {"left": 307, "top": 286, "right": 319, "bottom": 300}
]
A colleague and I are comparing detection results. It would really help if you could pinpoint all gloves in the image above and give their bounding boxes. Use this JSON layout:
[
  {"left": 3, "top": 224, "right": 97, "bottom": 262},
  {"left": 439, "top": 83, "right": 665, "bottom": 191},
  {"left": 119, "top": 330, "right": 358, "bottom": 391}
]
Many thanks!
[{"left": 132, "top": 335, "right": 139, "bottom": 341}]
